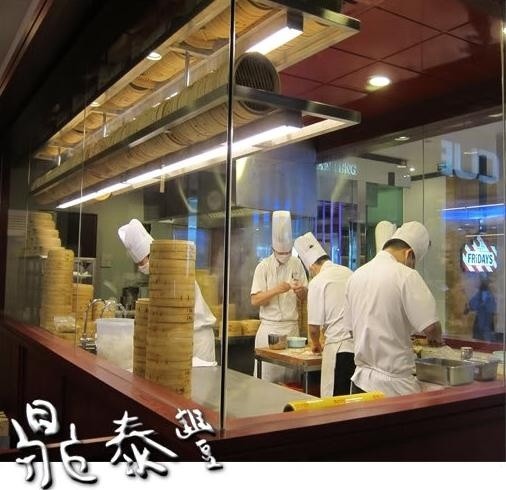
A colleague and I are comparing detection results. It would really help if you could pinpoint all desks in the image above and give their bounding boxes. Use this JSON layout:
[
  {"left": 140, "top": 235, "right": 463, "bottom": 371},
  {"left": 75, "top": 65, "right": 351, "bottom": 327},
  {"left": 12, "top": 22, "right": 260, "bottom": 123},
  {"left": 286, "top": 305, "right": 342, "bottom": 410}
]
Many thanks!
[{"left": 252, "top": 342, "right": 323, "bottom": 395}]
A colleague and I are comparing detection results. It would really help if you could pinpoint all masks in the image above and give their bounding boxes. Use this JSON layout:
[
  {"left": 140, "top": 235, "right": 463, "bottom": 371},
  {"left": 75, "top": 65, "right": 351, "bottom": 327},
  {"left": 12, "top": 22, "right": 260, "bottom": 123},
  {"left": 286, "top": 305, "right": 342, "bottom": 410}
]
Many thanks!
[
  {"left": 136, "top": 263, "right": 150, "bottom": 277},
  {"left": 275, "top": 253, "right": 292, "bottom": 263}
]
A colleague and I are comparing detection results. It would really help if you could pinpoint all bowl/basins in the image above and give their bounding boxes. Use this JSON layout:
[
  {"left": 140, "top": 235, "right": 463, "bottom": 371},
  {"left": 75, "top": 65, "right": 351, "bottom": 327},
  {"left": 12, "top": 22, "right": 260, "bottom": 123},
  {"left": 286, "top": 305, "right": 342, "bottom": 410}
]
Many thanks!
[
  {"left": 287, "top": 336, "right": 307, "bottom": 348},
  {"left": 267, "top": 334, "right": 287, "bottom": 349}
]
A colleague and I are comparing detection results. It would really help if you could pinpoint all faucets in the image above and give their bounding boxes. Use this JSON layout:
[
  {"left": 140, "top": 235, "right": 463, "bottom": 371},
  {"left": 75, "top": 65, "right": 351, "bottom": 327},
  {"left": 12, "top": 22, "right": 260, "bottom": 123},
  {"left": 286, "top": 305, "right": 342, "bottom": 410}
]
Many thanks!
[
  {"left": 98, "top": 300, "right": 127, "bottom": 319},
  {"left": 80, "top": 297, "right": 111, "bottom": 349}
]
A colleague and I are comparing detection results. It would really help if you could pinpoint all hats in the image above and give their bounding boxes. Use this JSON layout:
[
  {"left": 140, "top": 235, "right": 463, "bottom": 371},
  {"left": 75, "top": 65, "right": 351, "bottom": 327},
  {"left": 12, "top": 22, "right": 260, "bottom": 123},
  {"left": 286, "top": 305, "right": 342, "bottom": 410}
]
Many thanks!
[
  {"left": 117, "top": 218, "right": 157, "bottom": 264},
  {"left": 272, "top": 210, "right": 292, "bottom": 252},
  {"left": 375, "top": 220, "right": 397, "bottom": 253},
  {"left": 381, "top": 221, "right": 430, "bottom": 271},
  {"left": 293, "top": 232, "right": 328, "bottom": 272}
]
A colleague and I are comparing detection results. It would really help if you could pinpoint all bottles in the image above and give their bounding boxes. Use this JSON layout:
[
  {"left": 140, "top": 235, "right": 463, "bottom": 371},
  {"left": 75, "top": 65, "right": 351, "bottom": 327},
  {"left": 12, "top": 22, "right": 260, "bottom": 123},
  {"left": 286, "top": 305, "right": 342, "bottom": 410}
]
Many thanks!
[{"left": 461, "top": 346, "right": 473, "bottom": 361}]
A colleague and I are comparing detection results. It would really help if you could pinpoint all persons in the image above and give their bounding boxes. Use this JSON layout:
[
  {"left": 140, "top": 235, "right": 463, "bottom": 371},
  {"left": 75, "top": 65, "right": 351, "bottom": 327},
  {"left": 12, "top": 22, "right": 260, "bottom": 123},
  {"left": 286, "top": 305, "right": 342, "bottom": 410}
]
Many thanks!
[
  {"left": 136, "top": 253, "right": 216, "bottom": 363},
  {"left": 250, "top": 247, "right": 309, "bottom": 383},
  {"left": 307, "top": 255, "right": 354, "bottom": 399},
  {"left": 343, "top": 239, "right": 441, "bottom": 398}
]
